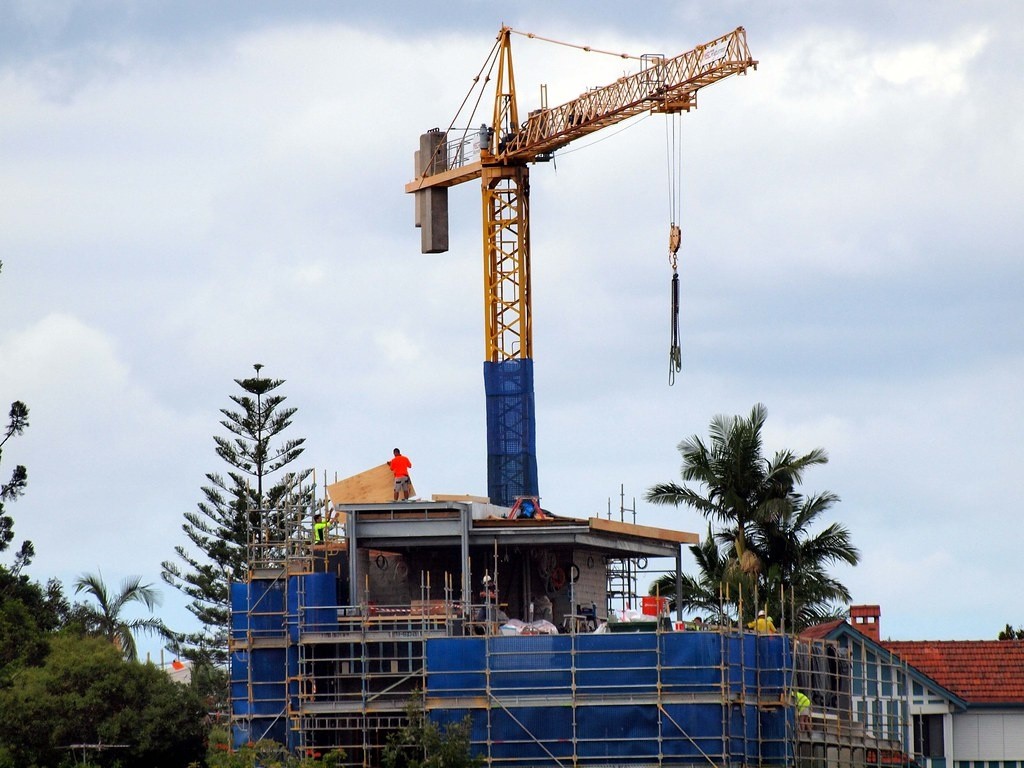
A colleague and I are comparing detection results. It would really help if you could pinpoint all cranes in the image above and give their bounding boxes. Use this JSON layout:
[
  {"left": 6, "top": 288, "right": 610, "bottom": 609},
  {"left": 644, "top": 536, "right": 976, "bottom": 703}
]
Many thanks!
[{"left": 399, "top": 21, "right": 761, "bottom": 526}]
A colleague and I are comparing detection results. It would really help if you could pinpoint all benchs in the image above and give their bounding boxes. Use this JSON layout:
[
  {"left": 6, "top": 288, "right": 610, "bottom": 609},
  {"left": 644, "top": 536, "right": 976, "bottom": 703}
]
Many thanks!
[{"left": 464, "top": 621, "right": 506, "bottom": 635}]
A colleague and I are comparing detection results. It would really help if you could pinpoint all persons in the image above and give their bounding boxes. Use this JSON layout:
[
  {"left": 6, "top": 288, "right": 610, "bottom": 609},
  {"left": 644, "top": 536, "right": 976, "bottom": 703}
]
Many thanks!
[
  {"left": 748, "top": 610, "right": 778, "bottom": 634},
  {"left": 313, "top": 507, "right": 340, "bottom": 545},
  {"left": 791, "top": 689, "right": 812, "bottom": 731},
  {"left": 387, "top": 448, "right": 412, "bottom": 502},
  {"left": 479, "top": 579, "right": 499, "bottom": 620},
  {"left": 694, "top": 617, "right": 702, "bottom": 627}
]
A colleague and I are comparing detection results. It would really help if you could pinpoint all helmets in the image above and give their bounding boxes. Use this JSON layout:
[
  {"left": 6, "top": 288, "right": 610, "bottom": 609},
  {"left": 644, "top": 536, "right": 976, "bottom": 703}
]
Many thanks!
[
  {"left": 758, "top": 610, "right": 765, "bottom": 616},
  {"left": 483, "top": 575, "right": 492, "bottom": 584}
]
